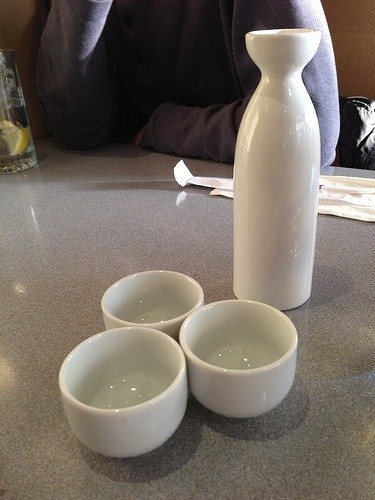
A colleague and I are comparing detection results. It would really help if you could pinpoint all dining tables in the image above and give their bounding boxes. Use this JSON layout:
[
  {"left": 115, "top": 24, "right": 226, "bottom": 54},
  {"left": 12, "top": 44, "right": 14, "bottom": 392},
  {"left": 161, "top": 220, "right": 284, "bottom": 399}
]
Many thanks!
[{"left": 1, "top": 133, "right": 375, "bottom": 500}]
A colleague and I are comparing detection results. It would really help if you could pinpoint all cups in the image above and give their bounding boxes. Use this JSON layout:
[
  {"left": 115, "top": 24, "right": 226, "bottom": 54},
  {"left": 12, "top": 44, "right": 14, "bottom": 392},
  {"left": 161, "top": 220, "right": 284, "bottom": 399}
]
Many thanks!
[
  {"left": 101, "top": 270, "right": 205, "bottom": 341},
  {"left": 0, "top": 48, "right": 36, "bottom": 175},
  {"left": 58, "top": 327, "right": 187, "bottom": 458},
  {"left": 178, "top": 299, "right": 299, "bottom": 418}
]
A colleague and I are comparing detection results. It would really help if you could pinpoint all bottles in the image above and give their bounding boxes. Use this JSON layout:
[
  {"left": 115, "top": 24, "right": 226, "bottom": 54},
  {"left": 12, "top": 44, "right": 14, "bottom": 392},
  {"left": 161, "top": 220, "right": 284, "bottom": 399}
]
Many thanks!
[{"left": 233, "top": 28, "right": 321, "bottom": 310}]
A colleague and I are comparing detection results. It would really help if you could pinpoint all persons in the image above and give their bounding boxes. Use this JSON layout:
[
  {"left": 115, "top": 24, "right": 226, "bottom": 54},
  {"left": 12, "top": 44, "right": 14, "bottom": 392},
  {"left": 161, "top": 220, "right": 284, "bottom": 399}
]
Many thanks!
[{"left": 33, "top": 0, "right": 342, "bottom": 169}]
